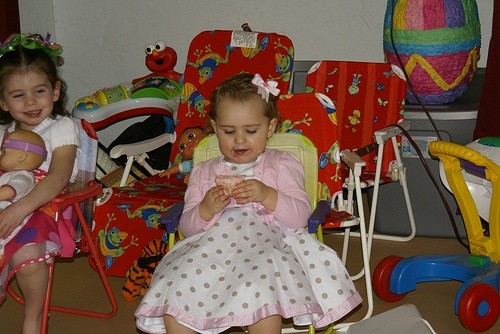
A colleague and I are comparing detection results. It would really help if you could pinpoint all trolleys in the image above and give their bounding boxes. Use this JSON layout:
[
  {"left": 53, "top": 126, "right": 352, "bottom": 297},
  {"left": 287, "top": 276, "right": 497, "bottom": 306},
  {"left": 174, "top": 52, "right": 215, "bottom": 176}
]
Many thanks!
[{"left": 371, "top": 133, "right": 500, "bottom": 334}]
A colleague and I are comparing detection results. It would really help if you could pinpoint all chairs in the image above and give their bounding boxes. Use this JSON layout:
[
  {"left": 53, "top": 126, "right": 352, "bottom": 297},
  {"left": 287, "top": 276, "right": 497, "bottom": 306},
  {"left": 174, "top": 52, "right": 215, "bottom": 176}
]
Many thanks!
[{"left": 0, "top": 29, "right": 406, "bottom": 334}]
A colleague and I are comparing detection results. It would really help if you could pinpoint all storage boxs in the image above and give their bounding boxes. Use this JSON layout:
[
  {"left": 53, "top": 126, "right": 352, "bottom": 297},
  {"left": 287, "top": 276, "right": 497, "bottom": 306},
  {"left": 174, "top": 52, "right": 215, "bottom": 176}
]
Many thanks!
[{"left": 367, "top": 104, "right": 479, "bottom": 239}]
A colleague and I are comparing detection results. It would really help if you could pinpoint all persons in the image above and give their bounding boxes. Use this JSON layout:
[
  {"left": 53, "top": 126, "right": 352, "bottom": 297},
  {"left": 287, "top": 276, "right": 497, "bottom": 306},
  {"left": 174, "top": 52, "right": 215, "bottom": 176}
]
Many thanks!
[
  {"left": 0, "top": 44, "right": 79, "bottom": 334},
  {"left": 158, "top": 73, "right": 311, "bottom": 334},
  {"left": 0, "top": 130, "right": 44, "bottom": 235}
]
